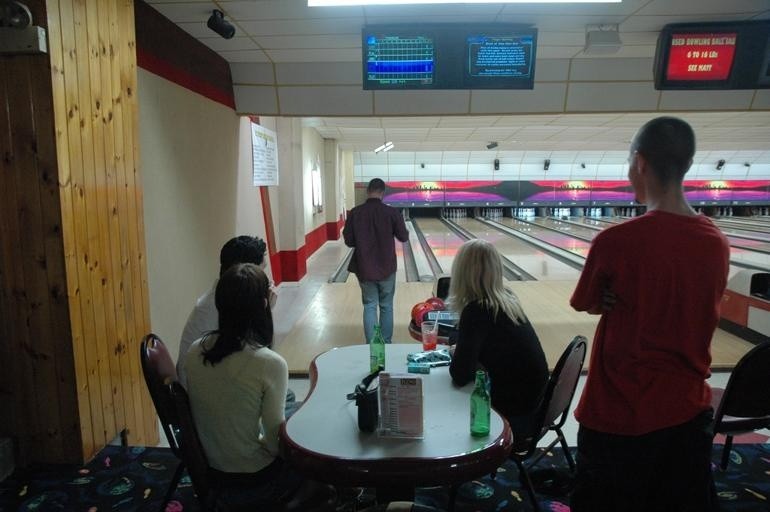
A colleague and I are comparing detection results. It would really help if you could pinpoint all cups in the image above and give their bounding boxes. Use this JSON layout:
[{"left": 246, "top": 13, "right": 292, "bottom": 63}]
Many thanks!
[{"left": 421, "top": 321, "right": 438, "bottom": 351}]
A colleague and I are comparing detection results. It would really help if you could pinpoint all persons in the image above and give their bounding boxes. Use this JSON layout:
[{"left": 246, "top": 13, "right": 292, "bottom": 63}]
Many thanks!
[
  {"left": 342, "top": 177, "right": 409, "bottom": 344},
  {"left": 176, "top": 234, "right": 296, "bottom": 402},
  {"left": 447, "top": 239, "right": 551, "bottom": 449},
  {"left": 570, "top": 116, "right": 730, "bottom": 512},
  {"left": 183, "top": 262, "right": 302, "bottom": 496}
]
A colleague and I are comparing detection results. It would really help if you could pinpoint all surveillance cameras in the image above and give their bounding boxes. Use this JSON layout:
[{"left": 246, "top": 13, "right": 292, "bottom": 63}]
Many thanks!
[
  {"left": 487, "top": 143, "right": 498, "bottom": 149},
  {"left": 207, "top": 14, "right": 235, "bottom": 39}
]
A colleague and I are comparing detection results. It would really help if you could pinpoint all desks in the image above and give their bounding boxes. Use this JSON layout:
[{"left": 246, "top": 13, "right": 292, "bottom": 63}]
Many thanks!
[{"left": 279, "top": 343, "right": 514, "bottom": 510}]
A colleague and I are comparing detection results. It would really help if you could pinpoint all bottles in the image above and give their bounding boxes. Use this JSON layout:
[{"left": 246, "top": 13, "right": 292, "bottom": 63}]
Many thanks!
[
  {"left": 469, "top": 370, "right": 491, "bottom": 438},
  {"left": 369, "top": 323, "right": 386, "bottom": 373}
]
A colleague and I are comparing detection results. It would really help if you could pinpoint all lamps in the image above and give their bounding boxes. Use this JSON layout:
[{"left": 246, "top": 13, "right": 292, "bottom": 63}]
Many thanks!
[{"left": 583, "top": 21, "right": 622, "bottom": 55}]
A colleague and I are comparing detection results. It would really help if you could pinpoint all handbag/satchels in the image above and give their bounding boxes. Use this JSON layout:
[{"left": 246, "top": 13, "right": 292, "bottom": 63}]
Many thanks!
[{"left": 348, "top": 366, "right": 384, "bottom": 434}]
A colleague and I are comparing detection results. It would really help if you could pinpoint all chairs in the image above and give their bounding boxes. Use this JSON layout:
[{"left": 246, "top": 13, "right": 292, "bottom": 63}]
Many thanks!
[
  {"left": 448, "top": 334, "right": 588, "bottom": 509},
  {"left": 141, "top": 333, "right": 199, "bottom": 507},
  {"left": 708, "top": 339, "right": 770, "bottom": 474},
  {"left": 168, "top": 380, "right": 334, "bottom": 509}
]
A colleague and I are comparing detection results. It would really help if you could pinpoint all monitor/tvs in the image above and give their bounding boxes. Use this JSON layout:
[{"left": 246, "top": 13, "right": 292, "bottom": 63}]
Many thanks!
[
  {"left": 363, "top": 26, "right": 444, "bottom": 90},
  {"left": 459, "top": 25, "right": 538, "bottom": 90},
  {"left": 655, "top": 22, "right": 749, "bottom": 90}
]
